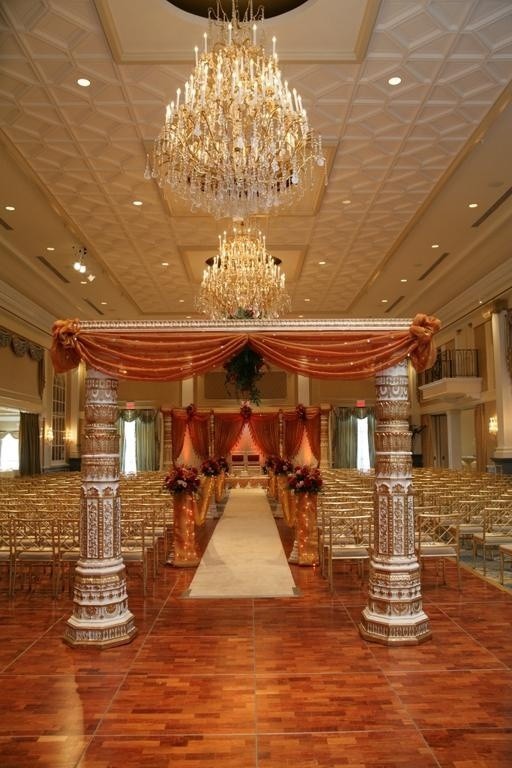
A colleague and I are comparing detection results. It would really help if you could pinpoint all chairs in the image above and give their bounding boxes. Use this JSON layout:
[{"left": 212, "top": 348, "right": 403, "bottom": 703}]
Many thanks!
[
  {"left": 0, "top": 471, "right": 175, "bottom": 602},
  {"left": 315, "top": 467, "right": 512, "bottom": 593}
]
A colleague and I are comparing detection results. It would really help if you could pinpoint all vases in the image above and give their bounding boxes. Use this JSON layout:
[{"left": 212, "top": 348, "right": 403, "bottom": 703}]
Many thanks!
[
  {"left": 268, "top": 471, "right": 285, "bottom": 518},
  {"left": 288, "top": 492, "right": 317, "bottom": 565},
  {"left": 166, "top": 494, "right": 198, "bottom": 568},
  {"left": 205, "top": 472, "right": 225, "bottom": 519}
]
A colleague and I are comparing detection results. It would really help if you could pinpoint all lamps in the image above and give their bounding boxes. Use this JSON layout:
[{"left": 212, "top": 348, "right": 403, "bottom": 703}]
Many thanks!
[
  {"left": 193, "top": 216, "right": 293, "bottom": 323},
  {"left": 74, "top": 246, "right": 88, "bottom": 275},
  {"left": 144, "top": 0, "right": 325, "bottom": 224}
]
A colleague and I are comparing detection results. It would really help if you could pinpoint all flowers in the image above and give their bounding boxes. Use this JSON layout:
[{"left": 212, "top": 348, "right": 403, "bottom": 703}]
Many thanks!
[
  {"left": 200, "top": 456, "right": 228, "bottom": 476},
  {"left": 163, "top": 464, "right": 201, "bottom": 495},
  {"left": 221, "top": 341, "right": 269, "bottom": 409},
  {"left": 262, "top": 454, "right": 293, "bottom": 475},
  {"left": 288, "top": 464, "right": 323, "bottom": 494}
]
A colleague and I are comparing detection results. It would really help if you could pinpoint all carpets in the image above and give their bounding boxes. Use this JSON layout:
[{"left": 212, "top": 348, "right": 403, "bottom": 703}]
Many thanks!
[{"left": 178, "top": 487, "right": 305, "bottom": 598}]
